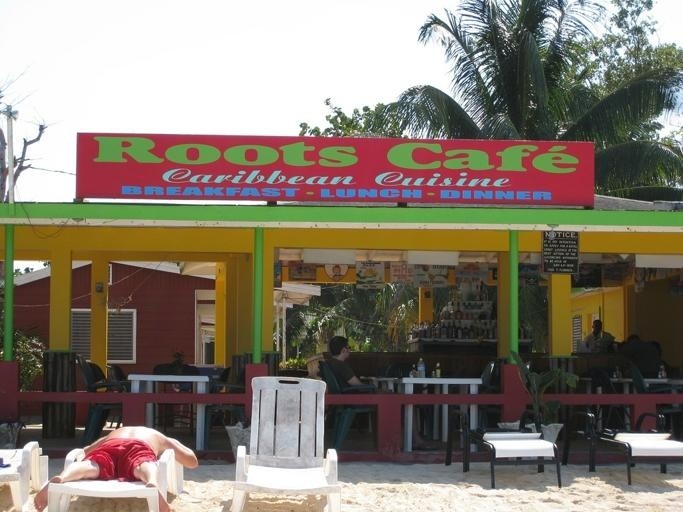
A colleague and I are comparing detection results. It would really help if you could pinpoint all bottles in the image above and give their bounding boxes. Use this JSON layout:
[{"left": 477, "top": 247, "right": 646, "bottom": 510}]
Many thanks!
[
  {"left": 411, "top": 357, "right": 441, "bottom": 378},
  {"left": 410, "top": 281, "right": 498, "bottom": 340}
]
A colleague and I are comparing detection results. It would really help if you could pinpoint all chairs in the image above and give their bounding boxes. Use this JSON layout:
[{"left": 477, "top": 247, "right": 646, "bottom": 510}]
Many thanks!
[
  {"left": 81, "top": 360, "right": 230, "bottom": 451},
  {"left": 231, "top": 377, "right": 338, "bottom": 511},
  {"left": 319, "top": 359, "right": 376, "bottom": 450}
]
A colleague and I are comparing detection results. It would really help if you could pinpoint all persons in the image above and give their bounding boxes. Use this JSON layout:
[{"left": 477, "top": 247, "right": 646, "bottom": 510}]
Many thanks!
[
  {"left": 584, "top": 319, "right": 612, "bottom": 353},
  {"left": 327, "top": 336, "right": 362, "bottom": 387},
  {"left": 34, "top": 424, "right": 199, "bottom": 512}
]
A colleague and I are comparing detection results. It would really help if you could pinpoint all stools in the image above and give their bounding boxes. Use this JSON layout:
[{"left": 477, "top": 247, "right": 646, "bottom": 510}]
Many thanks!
[
  {"left": 469, "top": 431, "right": 561, "bottom": 489},
  {"left": 587, "top": 431, "right": 683, "bottom": 484}
]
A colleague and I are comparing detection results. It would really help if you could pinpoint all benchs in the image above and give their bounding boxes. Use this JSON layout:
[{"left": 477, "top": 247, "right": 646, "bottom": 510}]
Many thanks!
[{"left": 46, "top": 448, "right": 183, "bottom": 511}]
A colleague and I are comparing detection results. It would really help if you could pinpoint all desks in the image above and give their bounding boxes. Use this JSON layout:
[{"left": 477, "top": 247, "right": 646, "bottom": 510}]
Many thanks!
[
  {"left": 576, "top": 378, "right": 683, "bottom": 431},
  {"left": 360, "top": 378, "right": 483, "bottom": 452}
]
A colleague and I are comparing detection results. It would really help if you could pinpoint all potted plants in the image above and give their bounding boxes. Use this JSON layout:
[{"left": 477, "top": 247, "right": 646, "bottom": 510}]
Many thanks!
[{"left": 496, "top": 350, "right": 580, "bottom": 445}]
